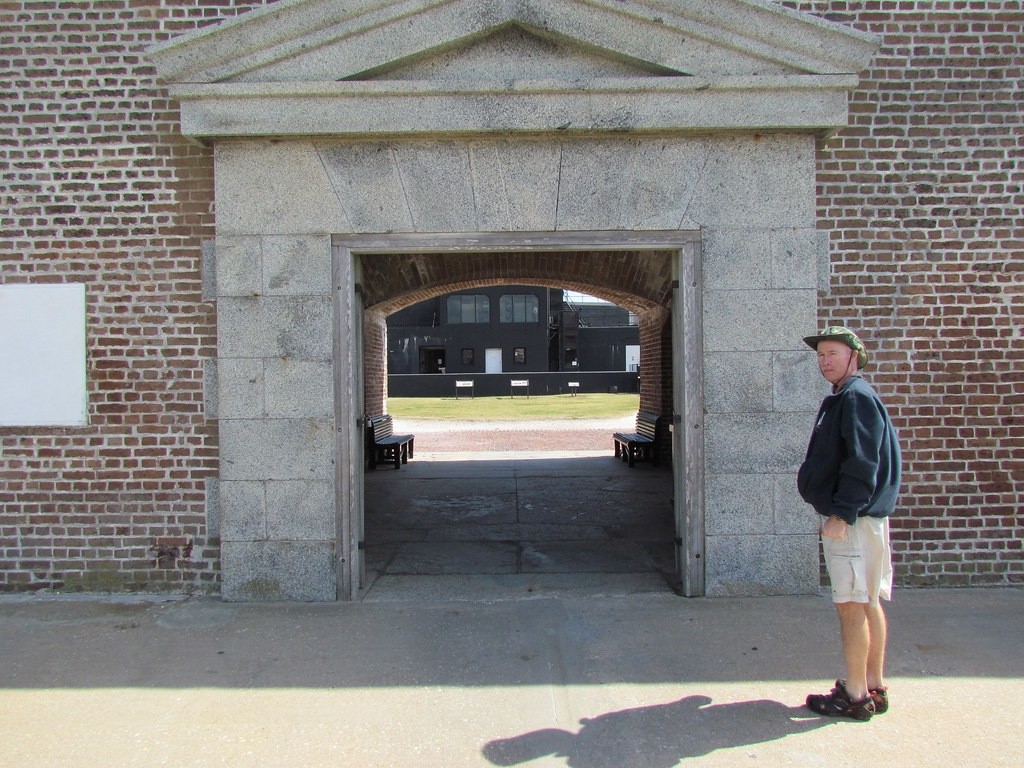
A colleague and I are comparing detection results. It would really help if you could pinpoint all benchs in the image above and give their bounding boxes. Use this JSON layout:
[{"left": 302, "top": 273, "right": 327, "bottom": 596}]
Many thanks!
[
  {"left": 613, "top": 410, "right": 662, "bottom": 469},
  {"left": 370, "top": 414, "right": 415, "bottom": 470}
]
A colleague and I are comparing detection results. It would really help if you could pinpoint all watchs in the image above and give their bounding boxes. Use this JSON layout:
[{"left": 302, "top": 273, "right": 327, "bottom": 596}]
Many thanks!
[{"left": 833, "top": 514, "right": 844, "bottom": 521}]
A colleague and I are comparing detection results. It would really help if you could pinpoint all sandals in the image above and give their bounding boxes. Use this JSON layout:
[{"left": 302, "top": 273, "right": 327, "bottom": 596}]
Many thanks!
[
  {"left": 836, "top": 680, "right": 889, "bottom": 714},
  {"left": 806, "top": 684, "right": 876, "bottom": 721}
]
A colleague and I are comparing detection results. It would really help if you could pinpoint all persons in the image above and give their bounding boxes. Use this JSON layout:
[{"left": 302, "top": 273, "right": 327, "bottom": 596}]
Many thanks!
[{"left": 797, "top": 326, "right": 902, "bottom": 719}]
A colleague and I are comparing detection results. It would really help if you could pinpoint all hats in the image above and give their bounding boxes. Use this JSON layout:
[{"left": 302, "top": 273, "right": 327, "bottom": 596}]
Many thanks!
[{"left": 802, "top": 326, "right": 868, "bottom": 370}]
